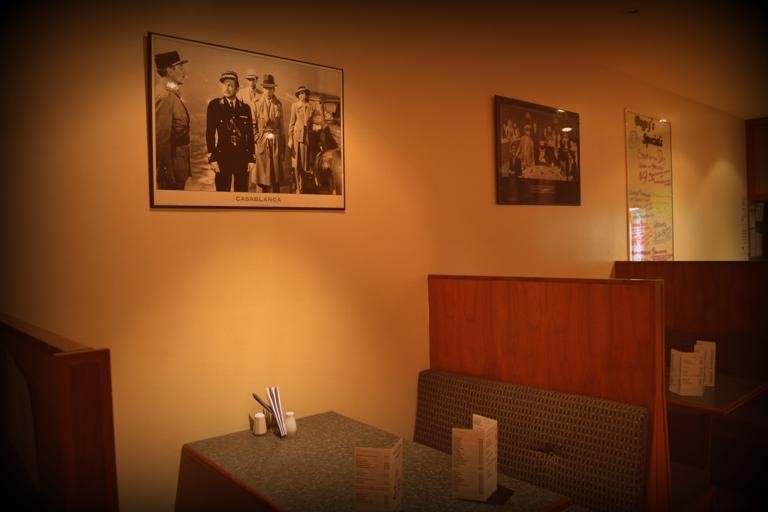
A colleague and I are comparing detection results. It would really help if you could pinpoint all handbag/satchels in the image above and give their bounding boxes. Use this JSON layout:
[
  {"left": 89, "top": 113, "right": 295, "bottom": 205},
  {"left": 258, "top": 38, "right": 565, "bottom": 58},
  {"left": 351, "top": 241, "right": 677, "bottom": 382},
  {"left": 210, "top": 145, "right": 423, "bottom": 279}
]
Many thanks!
[{"left": 313, "top": 121, "right": 338, "bottom": 154}]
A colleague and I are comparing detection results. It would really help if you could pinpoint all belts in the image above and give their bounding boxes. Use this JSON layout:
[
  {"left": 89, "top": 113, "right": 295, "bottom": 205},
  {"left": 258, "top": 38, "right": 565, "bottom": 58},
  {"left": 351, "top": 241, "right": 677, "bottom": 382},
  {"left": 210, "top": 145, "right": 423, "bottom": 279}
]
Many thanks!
[
  {"left": 171, "top": 136, "right": 190, "bottom": 147},
  {"left": 222, "top": 135, "right": 243, "bottom": 145}
]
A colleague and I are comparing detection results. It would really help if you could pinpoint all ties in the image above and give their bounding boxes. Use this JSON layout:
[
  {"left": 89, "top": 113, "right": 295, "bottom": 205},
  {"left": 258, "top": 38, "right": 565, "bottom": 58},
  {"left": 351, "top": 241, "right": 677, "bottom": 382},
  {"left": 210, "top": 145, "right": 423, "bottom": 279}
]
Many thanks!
[{"left": 230, "top": 100, "right": 235, "bottom": 112}]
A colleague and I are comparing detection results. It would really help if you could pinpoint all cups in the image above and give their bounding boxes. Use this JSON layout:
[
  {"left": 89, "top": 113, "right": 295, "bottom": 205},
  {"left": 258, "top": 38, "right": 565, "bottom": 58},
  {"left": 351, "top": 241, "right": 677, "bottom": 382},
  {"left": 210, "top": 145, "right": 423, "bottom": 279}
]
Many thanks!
[
  {"left": 285, "top": 411, "right": 297, "bottom": 432},
  {"left": 252, "top": 413, "right": 267, "bottom": 436}
]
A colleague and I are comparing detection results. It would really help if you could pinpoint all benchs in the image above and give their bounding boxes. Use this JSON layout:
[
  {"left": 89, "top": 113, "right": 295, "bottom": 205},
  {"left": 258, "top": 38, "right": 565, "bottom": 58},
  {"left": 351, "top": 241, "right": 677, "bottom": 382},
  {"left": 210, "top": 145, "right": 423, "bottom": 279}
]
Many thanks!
[{"left": 415, "top": 369, "right": 653, "bottom": 510}]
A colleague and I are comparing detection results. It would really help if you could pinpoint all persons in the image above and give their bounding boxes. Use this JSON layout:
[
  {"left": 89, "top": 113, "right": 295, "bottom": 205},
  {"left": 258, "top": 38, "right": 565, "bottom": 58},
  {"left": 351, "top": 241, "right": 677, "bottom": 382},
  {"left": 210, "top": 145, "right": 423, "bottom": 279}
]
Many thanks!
[
  {"left": 205, "top": 70, "right": 255, "bottom": 192},
  {"left": 249, "top": 73, "right": 286, "bottom": 193},
  {"left": 500, "top": 117, "right": 578, "bottom": 181},
  {"left": 236, "top": 72, "right": 263, "bottom": 191},
  {"left": 154, "top": 49, "right": 192, "bottom": 192},
  {"left": 288, "top": 84, "right": 322, "bottom": 194}
]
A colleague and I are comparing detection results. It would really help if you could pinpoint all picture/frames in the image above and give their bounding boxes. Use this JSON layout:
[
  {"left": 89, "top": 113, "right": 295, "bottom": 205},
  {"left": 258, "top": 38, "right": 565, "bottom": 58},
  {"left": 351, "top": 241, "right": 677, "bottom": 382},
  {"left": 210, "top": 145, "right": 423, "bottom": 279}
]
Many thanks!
[
  {"left": 147, "top": 29, "right": 344, "bottom": 211},
  {"left": 494, "top": 93, "right": 582, "bottom": 206}
]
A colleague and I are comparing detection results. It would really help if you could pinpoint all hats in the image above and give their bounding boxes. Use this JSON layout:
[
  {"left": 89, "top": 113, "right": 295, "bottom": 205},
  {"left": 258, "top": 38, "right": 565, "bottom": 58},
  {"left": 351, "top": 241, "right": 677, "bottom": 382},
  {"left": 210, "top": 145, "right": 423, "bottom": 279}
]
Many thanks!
[
  {"left": 220, "top": 71, "right": 238, "bottom": 82},
  {"left": 245, "top": 71, "right": 258, "bottom": 79},
  {"left": 296, "top": 86, "right": 310, "bottom": 97},
  {"left": 261, "top": 75, "right": 277, "bottom": 88},
  {"left": 154, "top": 51, "right": 188, "bottom": 68}
]
